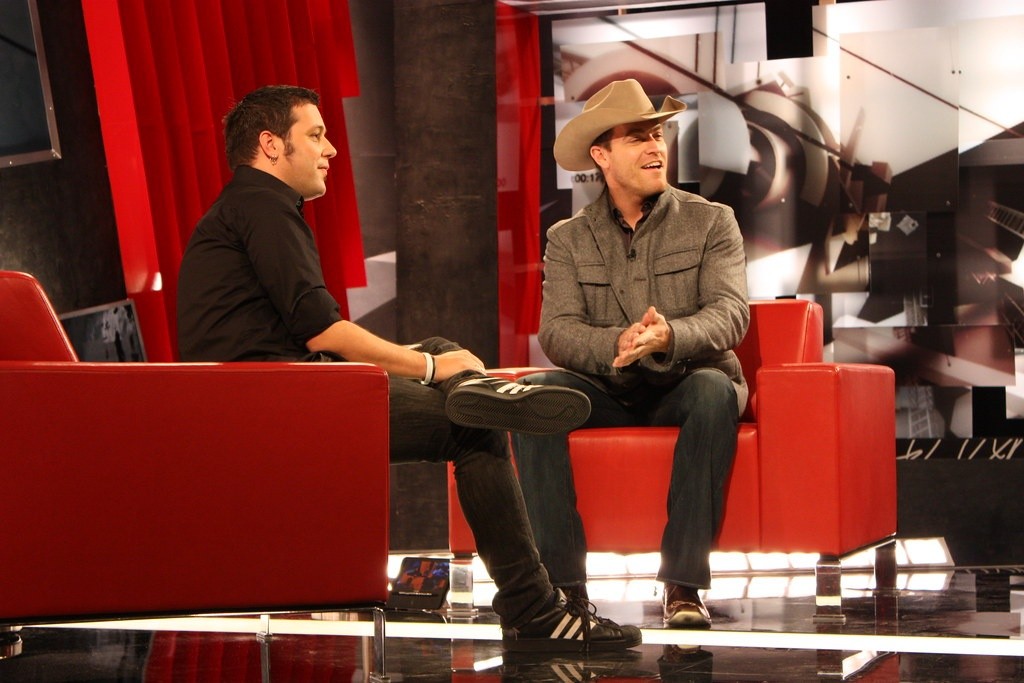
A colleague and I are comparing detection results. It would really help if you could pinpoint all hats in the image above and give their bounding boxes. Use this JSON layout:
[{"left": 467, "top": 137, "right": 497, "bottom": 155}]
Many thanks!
[{"left": 554, "top": 78, "right": 687, "bottom": 171}]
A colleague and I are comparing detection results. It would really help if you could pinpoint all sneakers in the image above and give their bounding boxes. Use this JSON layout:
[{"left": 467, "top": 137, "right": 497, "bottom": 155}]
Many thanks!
[
  {"left": 501, "top": 651, "right": 642, "bottom": 683},
  {"left": 445, "top": 374, "right": 592, "bottom": 436},
  {"left": 500, "top": 588, "right": 642, "bottom": 651}
]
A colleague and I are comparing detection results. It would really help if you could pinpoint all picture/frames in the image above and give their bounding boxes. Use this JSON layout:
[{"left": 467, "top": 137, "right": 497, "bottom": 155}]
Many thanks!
[
  {"left": 60, "top": 299, "right": 148, "bottom": 362},
  {"left": 0, "top": 0, "right": 62, "bottom": 169}
]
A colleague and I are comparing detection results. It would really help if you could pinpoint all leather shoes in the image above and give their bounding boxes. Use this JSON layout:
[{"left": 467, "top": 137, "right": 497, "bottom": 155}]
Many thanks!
[{"left": 663, "top": 581, "right": 711, "bottom": 630}]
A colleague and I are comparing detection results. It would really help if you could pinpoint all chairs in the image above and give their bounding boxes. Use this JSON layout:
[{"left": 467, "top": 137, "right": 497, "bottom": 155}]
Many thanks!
[
  {"left": 448, "top": 298, "right": 899, "bottom": 626},
  {"left": 0, "top": 270, "right": 391, "bottom": 683}
]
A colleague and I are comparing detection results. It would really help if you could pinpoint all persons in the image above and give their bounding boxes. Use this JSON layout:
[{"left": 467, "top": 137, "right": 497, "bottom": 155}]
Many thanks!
[
  {"left": 175, "top": 85, "right": 644, "bottom": 649},
  {"left": 511, "top": 79, "right": 750, "bottom": 628}
]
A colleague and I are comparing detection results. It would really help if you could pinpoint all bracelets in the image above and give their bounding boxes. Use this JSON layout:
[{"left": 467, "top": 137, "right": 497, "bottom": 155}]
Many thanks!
[{"left": 420, "top": 351, "right": 436, "bottom": 385}]
